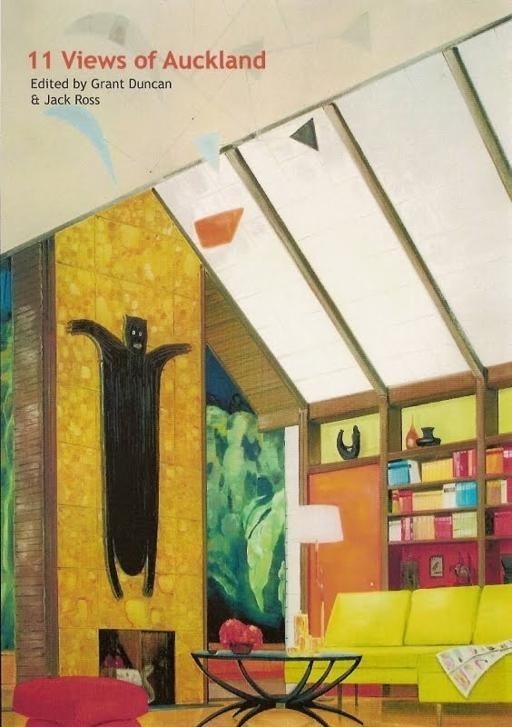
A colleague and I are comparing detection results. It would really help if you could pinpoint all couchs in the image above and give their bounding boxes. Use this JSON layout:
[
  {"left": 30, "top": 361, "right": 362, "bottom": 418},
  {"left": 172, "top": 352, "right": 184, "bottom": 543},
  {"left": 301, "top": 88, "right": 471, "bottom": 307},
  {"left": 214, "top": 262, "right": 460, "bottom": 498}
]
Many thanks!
[{"left": 284, "top": 583, "right": 512, "bottom": 726}]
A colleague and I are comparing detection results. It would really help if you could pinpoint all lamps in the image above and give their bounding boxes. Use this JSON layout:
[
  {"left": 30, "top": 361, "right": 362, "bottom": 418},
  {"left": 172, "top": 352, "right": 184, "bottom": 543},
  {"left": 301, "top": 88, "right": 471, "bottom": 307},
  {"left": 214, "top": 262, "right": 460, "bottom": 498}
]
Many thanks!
[{"left": 290, "top": 503, "right": 344, "bottom": 646}]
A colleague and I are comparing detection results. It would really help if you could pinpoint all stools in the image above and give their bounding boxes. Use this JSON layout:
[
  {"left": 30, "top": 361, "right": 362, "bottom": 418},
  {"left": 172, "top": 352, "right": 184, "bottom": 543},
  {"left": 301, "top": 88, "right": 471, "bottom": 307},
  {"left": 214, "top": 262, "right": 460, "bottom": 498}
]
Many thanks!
[{"left": 12, "top": 675, "right": 150, "bottom": 726}]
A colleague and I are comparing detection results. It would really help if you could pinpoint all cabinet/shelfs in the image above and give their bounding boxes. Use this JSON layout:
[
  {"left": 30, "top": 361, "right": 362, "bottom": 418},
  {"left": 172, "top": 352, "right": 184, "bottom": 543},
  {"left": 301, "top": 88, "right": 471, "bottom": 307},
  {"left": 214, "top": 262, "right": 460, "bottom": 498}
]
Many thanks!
[{"left": 304, "top": 373, "right": 511, "bottom": 635}]
